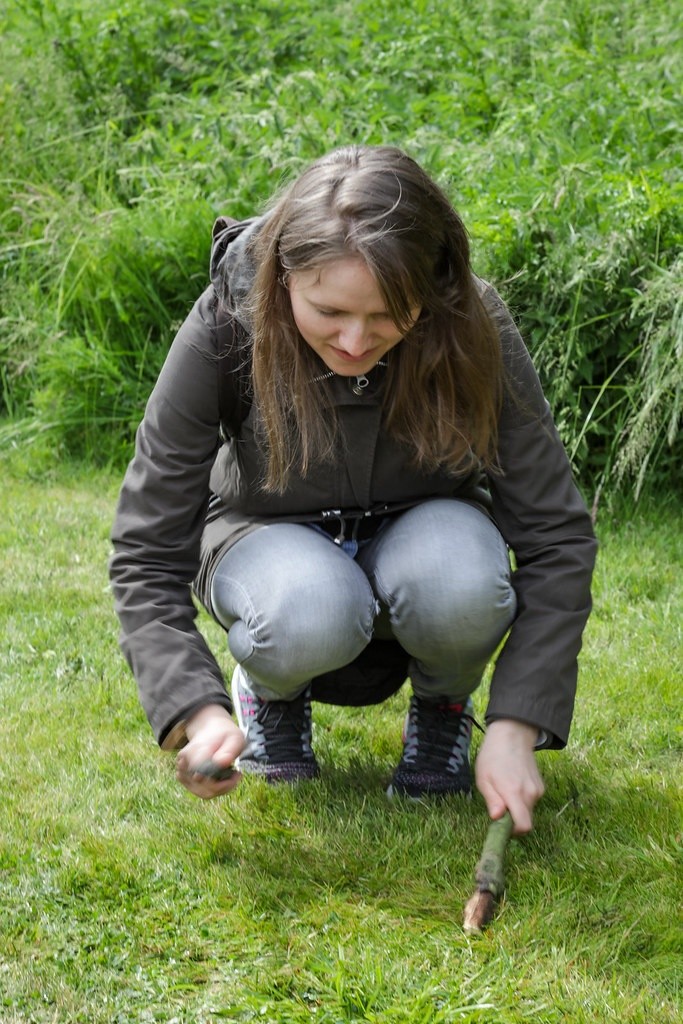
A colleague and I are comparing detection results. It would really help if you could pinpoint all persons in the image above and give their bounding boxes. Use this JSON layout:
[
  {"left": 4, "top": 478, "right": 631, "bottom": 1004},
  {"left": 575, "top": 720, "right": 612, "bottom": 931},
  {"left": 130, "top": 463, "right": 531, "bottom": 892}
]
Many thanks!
[{"left": 108, "top": 148, "right": 598, "bottom": 833}]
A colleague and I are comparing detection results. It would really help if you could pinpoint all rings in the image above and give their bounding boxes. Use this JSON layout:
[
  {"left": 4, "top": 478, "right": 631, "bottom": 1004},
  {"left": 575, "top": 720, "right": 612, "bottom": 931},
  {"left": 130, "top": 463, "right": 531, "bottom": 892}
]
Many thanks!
[{"left": 192, "top": 771, "right": 204, "bottom": 783}]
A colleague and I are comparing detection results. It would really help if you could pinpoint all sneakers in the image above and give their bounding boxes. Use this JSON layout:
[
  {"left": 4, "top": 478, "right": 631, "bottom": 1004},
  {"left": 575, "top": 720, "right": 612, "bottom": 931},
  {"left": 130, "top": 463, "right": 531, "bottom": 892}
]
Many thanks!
[
  {"left": 394, "top": 696, "right": 473, "bottom": 793},
  {"left": 231, "top": 664, "right": 320, "bottom": 781}
]
